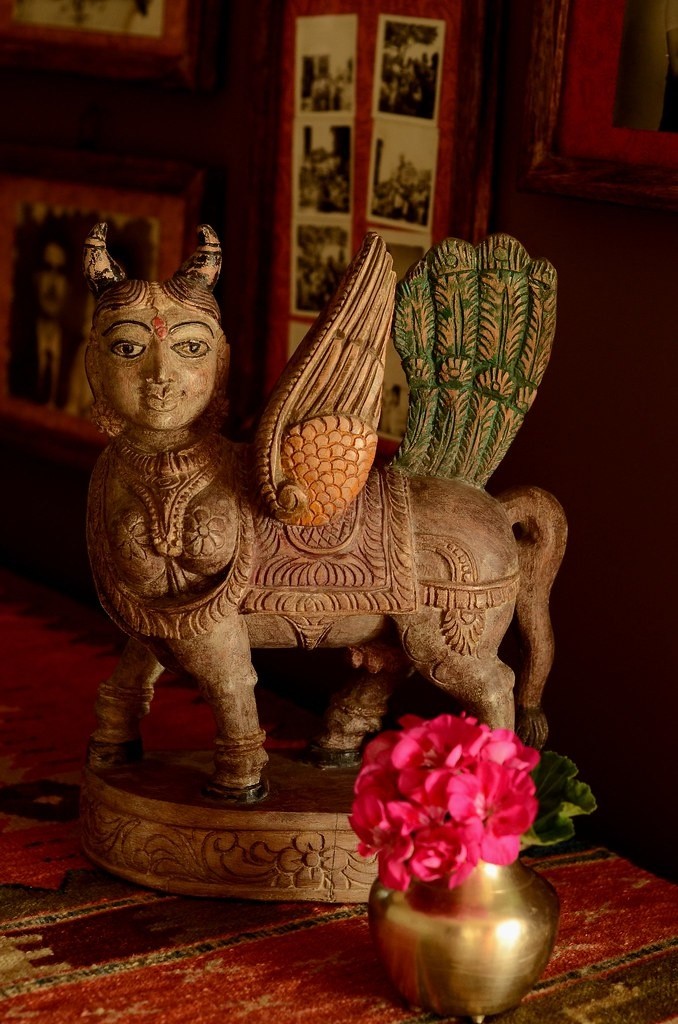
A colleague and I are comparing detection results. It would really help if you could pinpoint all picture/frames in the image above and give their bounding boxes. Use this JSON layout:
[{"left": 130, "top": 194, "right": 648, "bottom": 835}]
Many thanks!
[
  {"left": 236, "top": 0, "right": 509, "bottom": 476},
  {"left": 0, "top": 1, "right": 228, "bottom": 90},
  {"left": 519, "top": 1, "right": 677, "bottom": 213},
  {"left": 0, "top": 141, "right": 231, "bottom": 443}
]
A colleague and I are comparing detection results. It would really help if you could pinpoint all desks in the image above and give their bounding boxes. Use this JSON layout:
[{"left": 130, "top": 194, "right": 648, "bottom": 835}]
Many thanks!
[{"left": 0, "top": 569, "right": 678, "bottom": 1024}]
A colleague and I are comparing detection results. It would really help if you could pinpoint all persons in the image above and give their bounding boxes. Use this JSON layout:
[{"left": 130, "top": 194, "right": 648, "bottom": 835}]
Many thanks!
[
  {"left": 296, "top": 46, "right": 440, "bottom": 315},
  {"left": 10, "top": 238, "right": 81, "bottom": 412}
]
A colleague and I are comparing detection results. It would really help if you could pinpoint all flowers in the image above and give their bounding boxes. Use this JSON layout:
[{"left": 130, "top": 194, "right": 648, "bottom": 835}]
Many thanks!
[{"left": 344, "top": 711, "right": 598, "bottom": 891}]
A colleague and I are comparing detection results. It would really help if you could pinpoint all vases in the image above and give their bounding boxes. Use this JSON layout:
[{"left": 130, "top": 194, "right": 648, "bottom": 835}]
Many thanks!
[{"left": 366, "top": 857, "right": 561, "bottom": 1018}]
